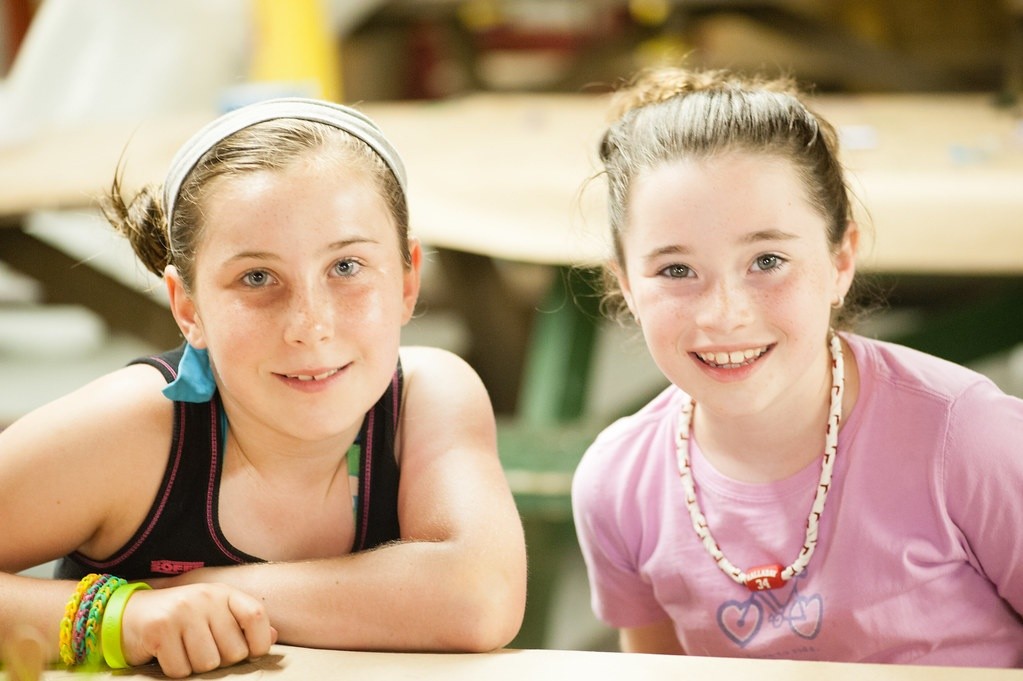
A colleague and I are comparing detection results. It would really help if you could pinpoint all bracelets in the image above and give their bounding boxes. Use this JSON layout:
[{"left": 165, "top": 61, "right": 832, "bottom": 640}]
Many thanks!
[
  {"left": 87, "top": 577, "right": 126, "bottom": 665},
  {"left": 60, "top": 574, "right": 100, "bottom": 667},
  {"left": 72, "top": 573, "right": 111, "bottom": 666},
  {"left": 101, "top": 583, "right": 150, "bottom": 669}
]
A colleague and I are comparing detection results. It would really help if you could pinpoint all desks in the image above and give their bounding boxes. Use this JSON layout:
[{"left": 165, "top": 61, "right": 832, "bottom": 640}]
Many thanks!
[{"left": 0, "top": 642, "right": 1022, "bottom": 680}]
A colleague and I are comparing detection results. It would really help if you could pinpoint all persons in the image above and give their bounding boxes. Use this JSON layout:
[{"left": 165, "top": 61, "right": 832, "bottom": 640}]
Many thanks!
[
  {"left": 570, "top": 71, "right": 1023, "bottom": 680},
  {"left": 0, "top": 100, "right": 527, "bottom": 678}
]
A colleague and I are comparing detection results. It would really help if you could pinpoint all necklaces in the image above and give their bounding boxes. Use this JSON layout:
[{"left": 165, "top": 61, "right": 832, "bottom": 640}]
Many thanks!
[{"left": 676, "top": 330, "right": 845, "bottom": 591}]
